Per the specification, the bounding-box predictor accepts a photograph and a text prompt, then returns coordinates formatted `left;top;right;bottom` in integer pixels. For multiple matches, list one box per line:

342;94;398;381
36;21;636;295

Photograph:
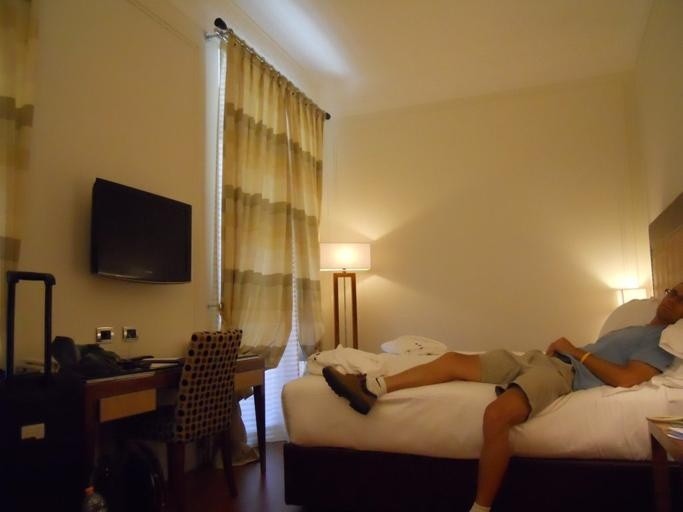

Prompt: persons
323;279;682;512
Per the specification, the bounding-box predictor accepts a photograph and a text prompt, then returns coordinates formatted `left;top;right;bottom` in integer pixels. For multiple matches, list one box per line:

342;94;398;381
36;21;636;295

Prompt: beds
281;193;683;512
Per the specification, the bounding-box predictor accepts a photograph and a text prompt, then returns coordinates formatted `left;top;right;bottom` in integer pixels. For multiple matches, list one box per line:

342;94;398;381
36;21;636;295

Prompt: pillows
600;296;683;389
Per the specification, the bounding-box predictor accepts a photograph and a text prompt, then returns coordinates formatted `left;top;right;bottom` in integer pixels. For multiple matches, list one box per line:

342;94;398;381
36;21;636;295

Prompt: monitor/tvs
90;178;192;285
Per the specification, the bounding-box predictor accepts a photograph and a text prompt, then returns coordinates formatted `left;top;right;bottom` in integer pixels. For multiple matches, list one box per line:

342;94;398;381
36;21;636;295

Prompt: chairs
113;328;242;512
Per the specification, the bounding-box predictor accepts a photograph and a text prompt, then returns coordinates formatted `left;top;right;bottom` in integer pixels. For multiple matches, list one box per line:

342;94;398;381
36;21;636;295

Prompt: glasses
665;288;683;304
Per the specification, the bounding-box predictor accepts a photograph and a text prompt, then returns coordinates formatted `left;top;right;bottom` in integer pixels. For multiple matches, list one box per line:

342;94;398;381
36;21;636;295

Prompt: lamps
319;243;371;349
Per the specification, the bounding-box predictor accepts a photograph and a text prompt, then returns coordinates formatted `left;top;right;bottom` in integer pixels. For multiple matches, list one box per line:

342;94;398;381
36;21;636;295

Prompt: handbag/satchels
79;342;150;375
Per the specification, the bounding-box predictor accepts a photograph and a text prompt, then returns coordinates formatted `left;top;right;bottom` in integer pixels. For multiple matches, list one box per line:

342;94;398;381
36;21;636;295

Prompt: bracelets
579;350;593;364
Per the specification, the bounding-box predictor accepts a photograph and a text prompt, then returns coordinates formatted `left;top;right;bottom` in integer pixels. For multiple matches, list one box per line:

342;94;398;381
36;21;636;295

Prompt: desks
0;358;269;512
648;414;683;512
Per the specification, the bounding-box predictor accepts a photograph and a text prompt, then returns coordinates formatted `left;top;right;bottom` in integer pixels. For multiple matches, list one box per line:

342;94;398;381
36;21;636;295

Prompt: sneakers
322;366;377;415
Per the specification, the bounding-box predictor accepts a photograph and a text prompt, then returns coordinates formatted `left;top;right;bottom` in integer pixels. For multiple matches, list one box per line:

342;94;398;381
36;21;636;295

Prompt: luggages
0;271;86;508
